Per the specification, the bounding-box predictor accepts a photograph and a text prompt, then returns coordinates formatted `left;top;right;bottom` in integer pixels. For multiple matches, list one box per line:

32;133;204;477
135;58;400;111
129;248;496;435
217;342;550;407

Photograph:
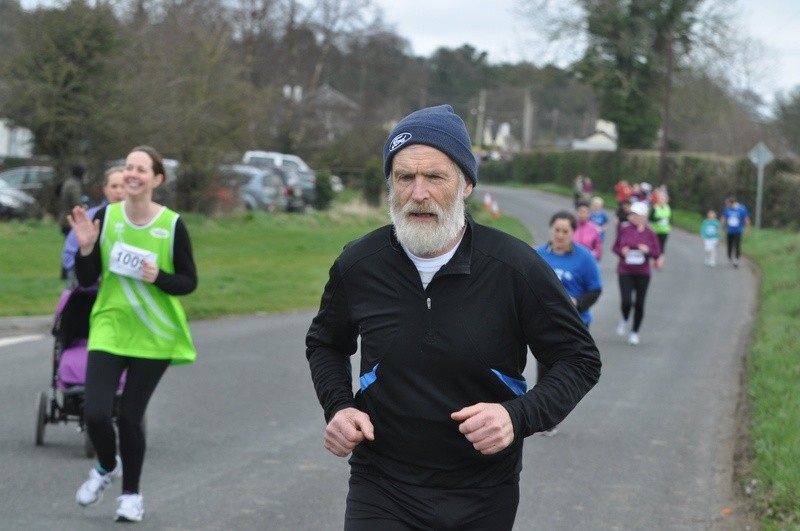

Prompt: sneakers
75;457;122;506
113;494;146;522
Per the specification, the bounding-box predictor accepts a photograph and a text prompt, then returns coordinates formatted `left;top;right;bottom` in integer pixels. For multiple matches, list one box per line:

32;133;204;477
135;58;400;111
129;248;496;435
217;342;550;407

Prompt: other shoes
629;331;638;345
617;318;629;336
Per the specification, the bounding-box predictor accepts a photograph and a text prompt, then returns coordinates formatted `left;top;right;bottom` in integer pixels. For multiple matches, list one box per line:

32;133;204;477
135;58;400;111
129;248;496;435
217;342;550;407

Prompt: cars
110;160;178;203
0;179;35;216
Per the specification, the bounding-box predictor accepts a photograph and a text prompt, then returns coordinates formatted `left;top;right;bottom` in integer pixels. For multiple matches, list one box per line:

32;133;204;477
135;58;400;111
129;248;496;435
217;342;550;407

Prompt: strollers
36;287;126;445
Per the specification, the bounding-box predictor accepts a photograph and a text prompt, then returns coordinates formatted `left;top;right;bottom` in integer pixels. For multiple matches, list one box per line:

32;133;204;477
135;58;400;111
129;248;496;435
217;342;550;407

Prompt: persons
720;195;750;268
305;103;602;531
61;146;198;520
532;175;609;333
614;178;672;271
611;202;661;345
700;210;721;268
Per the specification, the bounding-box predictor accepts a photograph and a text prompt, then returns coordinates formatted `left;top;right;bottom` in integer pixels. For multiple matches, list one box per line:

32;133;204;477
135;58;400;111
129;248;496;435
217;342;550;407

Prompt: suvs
216;148;344;213
0;166;55;198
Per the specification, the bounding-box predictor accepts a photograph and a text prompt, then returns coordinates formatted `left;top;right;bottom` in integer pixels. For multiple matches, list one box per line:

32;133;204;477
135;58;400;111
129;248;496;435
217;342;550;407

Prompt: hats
629;202;649;217
381;103;479;188
